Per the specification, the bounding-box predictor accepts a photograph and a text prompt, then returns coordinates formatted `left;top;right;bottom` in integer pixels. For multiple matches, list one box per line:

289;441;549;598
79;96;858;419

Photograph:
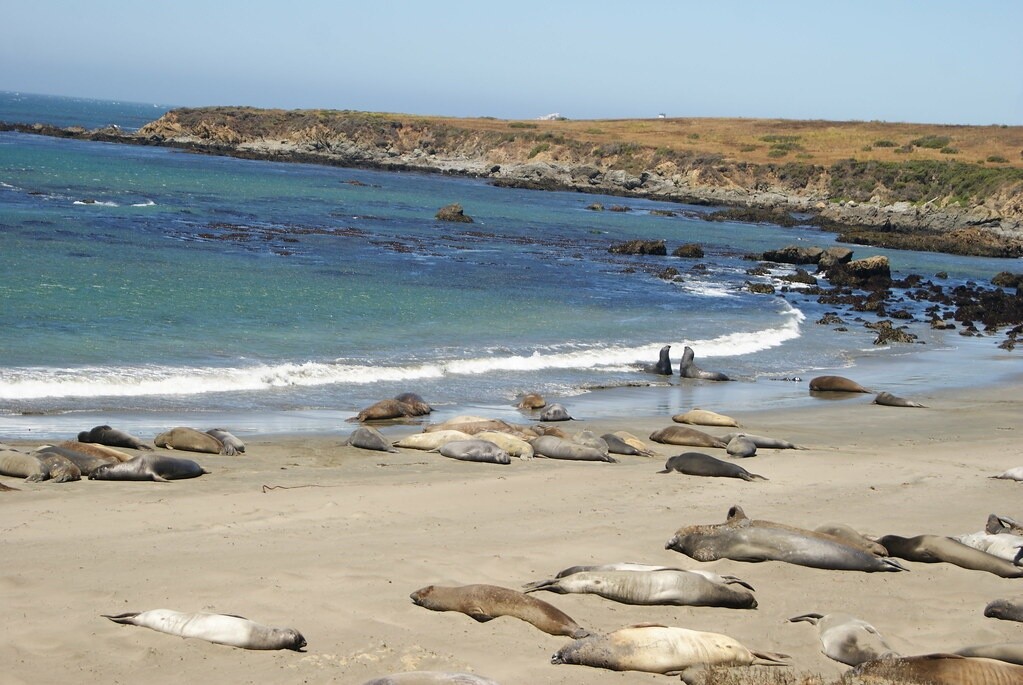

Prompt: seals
97;609;308;651
809;376;874;395
347;391;1023;685
643;346;673;375
1;422;246;485
680;346;736;382
874;392;930;408
